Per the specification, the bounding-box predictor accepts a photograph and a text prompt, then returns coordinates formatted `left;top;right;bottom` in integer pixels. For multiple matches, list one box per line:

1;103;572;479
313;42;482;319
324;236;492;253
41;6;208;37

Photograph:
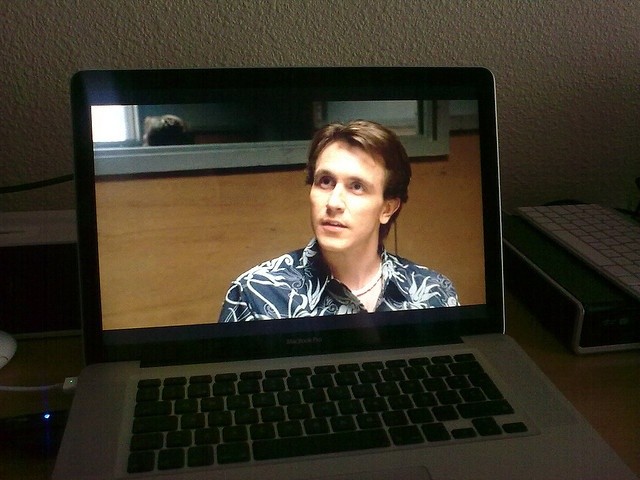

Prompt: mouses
0;330;20;372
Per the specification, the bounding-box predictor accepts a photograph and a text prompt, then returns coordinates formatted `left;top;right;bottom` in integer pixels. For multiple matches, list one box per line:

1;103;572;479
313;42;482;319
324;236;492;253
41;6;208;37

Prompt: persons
217;117;463;323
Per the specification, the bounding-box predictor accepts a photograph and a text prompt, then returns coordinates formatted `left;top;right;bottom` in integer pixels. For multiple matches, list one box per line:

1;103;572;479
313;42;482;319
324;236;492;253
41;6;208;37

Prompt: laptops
51;67;640;479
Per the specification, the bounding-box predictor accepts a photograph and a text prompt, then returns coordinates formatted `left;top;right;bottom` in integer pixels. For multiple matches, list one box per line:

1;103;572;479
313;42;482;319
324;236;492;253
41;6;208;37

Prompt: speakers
0;209;83;337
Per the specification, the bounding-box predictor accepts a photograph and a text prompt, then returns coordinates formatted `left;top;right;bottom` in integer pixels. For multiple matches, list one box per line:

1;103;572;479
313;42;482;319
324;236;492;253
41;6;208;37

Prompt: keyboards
516;202;639;301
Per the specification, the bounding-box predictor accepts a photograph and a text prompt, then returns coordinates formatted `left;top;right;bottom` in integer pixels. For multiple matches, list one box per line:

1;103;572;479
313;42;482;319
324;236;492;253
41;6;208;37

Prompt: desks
0;286;640;479
